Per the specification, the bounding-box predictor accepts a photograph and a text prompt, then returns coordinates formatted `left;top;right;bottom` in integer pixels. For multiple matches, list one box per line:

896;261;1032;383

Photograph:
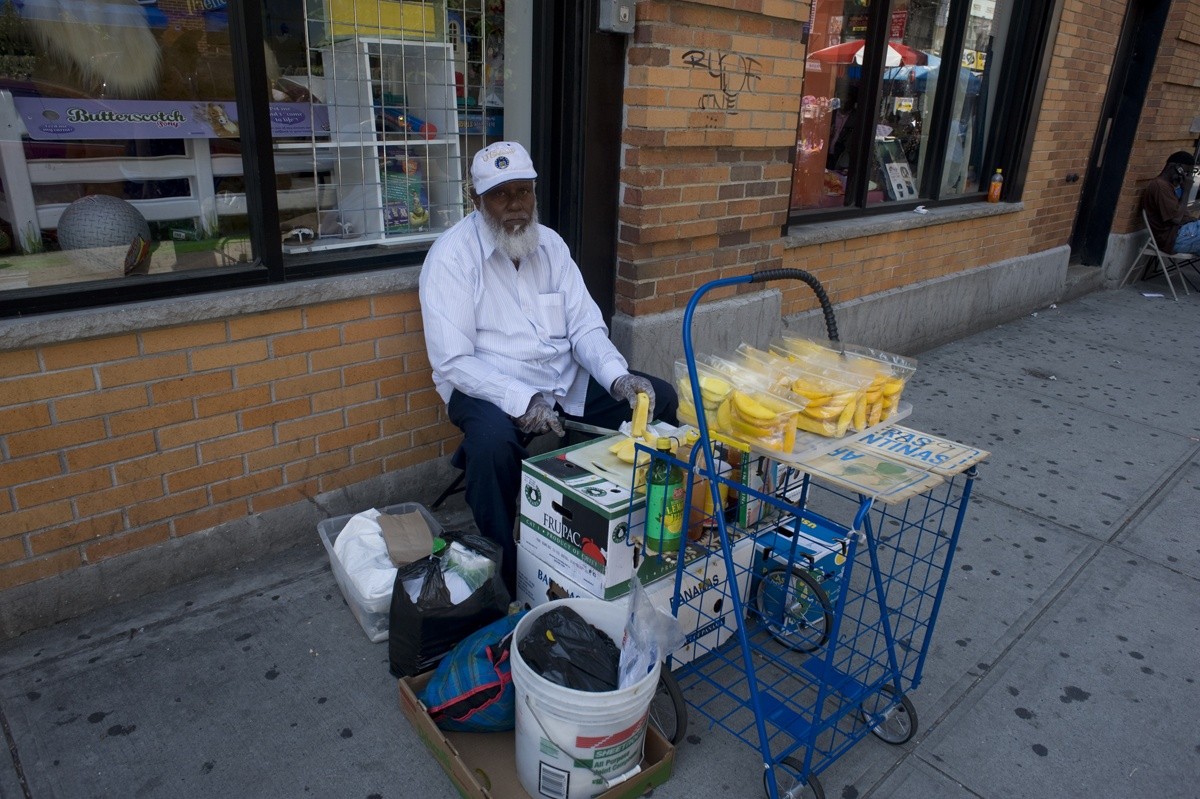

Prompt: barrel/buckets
509;598;662;799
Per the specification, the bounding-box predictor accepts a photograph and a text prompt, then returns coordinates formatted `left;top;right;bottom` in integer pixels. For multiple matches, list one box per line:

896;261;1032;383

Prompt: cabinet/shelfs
319;38;464;240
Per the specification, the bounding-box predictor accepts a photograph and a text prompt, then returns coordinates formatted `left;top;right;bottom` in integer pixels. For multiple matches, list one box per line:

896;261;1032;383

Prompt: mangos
611;338;902;464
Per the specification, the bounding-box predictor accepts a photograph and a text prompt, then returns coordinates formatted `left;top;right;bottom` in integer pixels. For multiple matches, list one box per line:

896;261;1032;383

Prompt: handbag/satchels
417;608;530;733
388;530;512;677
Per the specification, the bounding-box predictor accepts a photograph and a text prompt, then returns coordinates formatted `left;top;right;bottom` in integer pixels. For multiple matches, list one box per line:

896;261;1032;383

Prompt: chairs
1118;189;1200;301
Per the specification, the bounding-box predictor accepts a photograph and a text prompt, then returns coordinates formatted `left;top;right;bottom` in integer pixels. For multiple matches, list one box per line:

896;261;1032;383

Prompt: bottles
701;458;732;527
677;433;709;540
988;168;1003;203
644;437;688;553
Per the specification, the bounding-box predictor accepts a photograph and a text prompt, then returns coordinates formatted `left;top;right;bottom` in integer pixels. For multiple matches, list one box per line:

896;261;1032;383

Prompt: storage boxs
317;419;855;799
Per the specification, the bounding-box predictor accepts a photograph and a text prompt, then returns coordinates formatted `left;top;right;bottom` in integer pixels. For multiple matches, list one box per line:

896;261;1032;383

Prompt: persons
895;111;922;163
420;142;680;596
1141;151;1200;254
942;112;964;186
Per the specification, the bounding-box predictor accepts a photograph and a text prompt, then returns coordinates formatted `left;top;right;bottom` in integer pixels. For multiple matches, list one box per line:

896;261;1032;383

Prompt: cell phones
1176;166;1186;176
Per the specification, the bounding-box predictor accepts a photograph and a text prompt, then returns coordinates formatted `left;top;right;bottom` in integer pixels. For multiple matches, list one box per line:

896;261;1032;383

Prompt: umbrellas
846;51;981;110
807;40;928;124
